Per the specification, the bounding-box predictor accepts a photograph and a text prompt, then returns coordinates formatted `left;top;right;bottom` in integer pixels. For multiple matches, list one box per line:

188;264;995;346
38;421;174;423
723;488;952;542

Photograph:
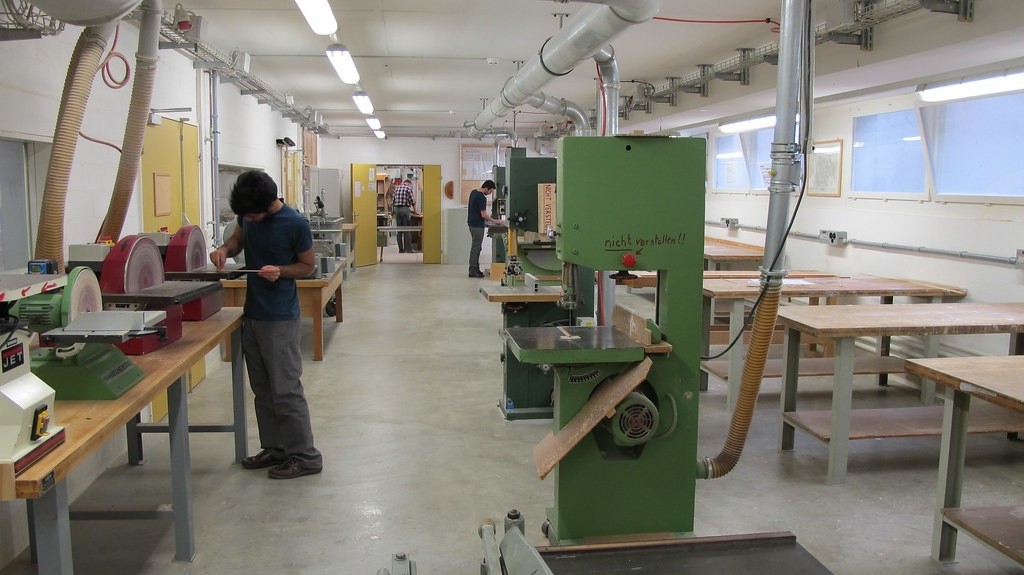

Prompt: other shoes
407;249;417;253
399;250;406;253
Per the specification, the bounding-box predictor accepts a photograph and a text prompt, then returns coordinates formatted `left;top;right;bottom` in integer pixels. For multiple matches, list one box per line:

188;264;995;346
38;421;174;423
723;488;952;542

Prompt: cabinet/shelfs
376;175;389;212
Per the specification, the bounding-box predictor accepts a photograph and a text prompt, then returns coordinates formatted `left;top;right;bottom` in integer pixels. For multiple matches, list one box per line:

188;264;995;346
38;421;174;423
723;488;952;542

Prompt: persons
210;171;323;480
466;180;506;278
394;179;418;255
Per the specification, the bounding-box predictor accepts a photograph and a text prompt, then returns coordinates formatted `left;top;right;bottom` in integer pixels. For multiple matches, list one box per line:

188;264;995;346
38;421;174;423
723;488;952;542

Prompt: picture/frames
806;139;843;197
154;173;171;217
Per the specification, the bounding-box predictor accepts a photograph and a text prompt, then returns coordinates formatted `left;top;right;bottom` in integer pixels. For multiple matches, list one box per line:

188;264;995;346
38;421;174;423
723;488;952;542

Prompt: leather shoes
268;461;322;480
241;450;287;469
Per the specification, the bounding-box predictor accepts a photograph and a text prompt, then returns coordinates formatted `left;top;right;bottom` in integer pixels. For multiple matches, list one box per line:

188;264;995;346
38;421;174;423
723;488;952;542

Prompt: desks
220;257;345;362
703;278;967;416
342;223;359;280
704;240;765;325
0;307;248;575
630;270;840;358
775;304;1024;486
411;213;423;249
377;226;423;264
905;355;1023;567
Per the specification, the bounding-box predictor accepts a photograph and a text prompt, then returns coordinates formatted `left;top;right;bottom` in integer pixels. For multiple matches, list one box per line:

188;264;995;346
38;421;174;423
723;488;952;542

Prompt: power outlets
725;218;738;230
828;231;847;246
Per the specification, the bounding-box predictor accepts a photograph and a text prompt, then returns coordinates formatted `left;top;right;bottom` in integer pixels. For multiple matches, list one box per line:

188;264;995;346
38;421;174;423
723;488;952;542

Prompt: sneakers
469;272;484;277
478;270;483;275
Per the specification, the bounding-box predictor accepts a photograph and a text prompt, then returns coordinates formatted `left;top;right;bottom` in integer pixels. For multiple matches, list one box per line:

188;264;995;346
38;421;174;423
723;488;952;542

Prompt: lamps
717;111;800;134
295;0;385;139
915;66;1024;102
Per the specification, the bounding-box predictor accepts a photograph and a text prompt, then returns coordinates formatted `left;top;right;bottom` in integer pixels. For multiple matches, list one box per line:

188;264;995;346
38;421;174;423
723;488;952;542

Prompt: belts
394;205;411;207
